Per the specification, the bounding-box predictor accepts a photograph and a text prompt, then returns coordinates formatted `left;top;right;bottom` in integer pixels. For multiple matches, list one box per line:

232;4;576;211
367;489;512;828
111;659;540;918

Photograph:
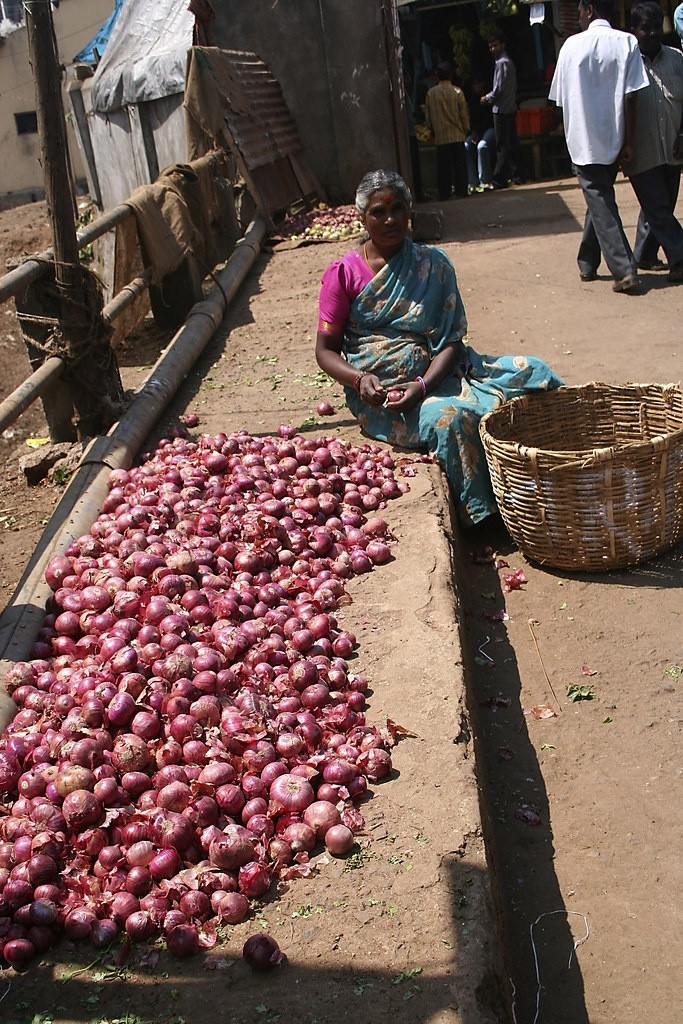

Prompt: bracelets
354;371;374;393
414;375;428;400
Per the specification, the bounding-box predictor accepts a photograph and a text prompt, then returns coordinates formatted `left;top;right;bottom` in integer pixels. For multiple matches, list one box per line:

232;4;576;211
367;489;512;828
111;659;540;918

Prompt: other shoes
467;182;492;195
667;271;683;282
612;273;641;292
578;271;597;280
638;259;669;270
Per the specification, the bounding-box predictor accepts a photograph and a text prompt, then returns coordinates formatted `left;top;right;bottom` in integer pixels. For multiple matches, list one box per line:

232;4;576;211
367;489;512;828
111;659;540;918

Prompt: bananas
502;2;519;17
413;123;430;141
449;23;476;75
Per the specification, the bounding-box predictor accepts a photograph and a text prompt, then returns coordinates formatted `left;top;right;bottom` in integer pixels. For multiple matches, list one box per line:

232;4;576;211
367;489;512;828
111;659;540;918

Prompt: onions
388;390;403;402
0;402;401;967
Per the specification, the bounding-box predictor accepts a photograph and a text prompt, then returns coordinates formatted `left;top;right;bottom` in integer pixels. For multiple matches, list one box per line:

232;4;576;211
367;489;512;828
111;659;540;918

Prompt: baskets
479;379;683;573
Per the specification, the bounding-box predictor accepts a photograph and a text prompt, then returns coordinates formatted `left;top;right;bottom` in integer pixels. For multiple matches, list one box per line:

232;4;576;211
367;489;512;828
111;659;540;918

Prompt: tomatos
279;205;366;239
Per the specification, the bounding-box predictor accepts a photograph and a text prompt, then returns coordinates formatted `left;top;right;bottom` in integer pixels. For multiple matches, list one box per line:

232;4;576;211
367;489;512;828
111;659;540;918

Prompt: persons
314;171;571;566
673;3;683;52
424;62;471;201
464;76;498;196
548;0;645;293
623;4;683;281
478;34;525;187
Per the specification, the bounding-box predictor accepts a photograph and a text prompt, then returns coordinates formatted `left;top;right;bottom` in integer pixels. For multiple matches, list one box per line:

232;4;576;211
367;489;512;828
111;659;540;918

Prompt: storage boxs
514;103;557;138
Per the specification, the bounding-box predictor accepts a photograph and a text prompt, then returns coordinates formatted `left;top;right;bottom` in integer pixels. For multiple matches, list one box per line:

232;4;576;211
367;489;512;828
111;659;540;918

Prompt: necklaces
357;237;401;277
